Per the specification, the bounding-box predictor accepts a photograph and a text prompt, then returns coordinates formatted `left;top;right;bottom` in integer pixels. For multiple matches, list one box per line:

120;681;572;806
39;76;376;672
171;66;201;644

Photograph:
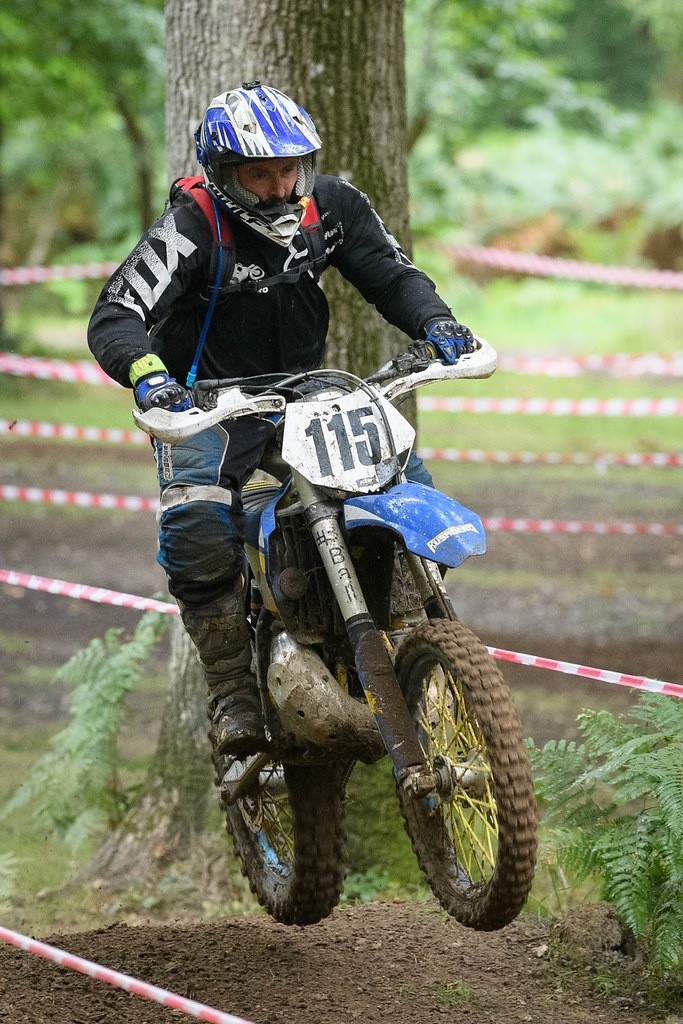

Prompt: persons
86;82;476;755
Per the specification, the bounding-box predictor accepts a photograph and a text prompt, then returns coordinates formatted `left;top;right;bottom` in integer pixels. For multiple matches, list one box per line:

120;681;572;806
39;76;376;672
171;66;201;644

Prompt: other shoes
207;677;264;751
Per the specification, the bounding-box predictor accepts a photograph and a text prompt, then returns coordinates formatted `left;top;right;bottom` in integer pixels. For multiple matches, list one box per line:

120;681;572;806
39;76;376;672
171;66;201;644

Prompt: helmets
195;82;322;245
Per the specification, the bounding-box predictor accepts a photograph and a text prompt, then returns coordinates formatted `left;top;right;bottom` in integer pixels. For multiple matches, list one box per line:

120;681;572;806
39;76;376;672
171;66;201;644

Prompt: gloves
424;316;477;366
135;373;192;414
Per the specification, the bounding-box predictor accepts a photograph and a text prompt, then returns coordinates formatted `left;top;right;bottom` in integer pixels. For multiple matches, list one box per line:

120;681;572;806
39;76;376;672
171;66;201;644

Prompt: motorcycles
131;335;539;931
